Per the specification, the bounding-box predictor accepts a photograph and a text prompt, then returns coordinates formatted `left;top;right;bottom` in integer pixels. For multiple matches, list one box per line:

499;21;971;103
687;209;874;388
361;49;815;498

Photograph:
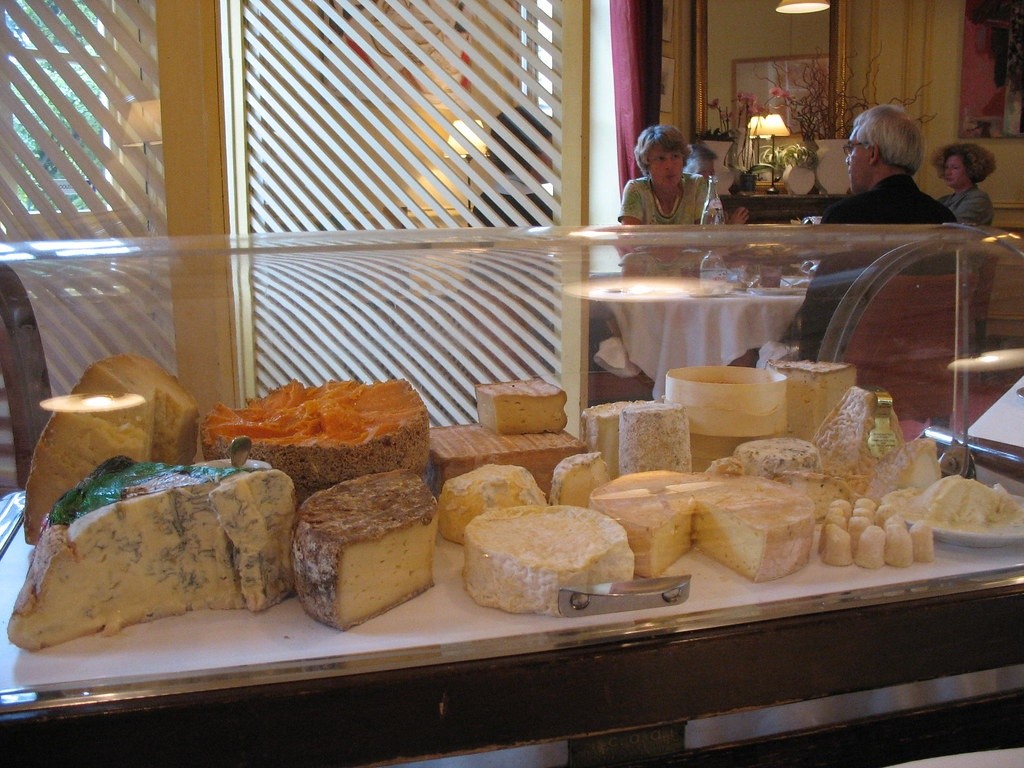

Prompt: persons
685;142;719;183
618;124;749;278
799;106;959;363
929;141;997;226
468;105;617;371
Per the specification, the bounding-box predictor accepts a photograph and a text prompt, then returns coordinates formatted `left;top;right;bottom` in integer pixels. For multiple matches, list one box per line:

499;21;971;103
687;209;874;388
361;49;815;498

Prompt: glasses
843;140;871;155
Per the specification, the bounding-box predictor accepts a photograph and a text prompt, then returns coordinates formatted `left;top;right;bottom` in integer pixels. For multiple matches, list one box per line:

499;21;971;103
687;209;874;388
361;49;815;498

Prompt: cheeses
5;354;941;653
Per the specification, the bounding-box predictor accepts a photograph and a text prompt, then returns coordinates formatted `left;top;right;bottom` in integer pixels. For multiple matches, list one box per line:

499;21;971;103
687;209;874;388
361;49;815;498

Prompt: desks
564;280;807;400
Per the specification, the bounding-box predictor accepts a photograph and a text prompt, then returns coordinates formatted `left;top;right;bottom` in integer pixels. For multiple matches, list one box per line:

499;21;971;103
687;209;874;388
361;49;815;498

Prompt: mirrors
693;0;844;194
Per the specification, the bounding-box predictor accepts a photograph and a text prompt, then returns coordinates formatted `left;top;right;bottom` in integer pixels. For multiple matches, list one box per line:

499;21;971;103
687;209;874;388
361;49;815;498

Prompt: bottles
699;174;724;225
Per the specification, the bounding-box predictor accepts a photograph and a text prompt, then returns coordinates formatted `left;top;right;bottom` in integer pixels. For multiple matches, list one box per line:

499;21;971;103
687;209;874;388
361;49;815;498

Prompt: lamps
444;120;488;223
124;100;162;146
775;0;830;15
747;116;768;182
762;113;791;193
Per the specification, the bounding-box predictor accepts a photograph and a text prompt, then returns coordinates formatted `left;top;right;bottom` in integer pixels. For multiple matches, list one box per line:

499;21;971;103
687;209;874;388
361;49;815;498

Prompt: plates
751;279;810;295
880;489;1024;549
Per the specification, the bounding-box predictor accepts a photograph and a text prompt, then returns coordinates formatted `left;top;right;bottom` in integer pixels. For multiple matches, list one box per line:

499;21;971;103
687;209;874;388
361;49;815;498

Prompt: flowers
707;87;793;172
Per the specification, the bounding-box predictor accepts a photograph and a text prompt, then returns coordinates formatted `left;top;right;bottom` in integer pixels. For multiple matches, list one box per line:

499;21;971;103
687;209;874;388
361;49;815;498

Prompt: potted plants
781;144;818;195
761;145;787;182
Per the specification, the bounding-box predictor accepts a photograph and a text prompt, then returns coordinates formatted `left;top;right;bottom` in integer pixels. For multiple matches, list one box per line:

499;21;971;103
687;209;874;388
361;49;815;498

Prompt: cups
758;266;783;288
